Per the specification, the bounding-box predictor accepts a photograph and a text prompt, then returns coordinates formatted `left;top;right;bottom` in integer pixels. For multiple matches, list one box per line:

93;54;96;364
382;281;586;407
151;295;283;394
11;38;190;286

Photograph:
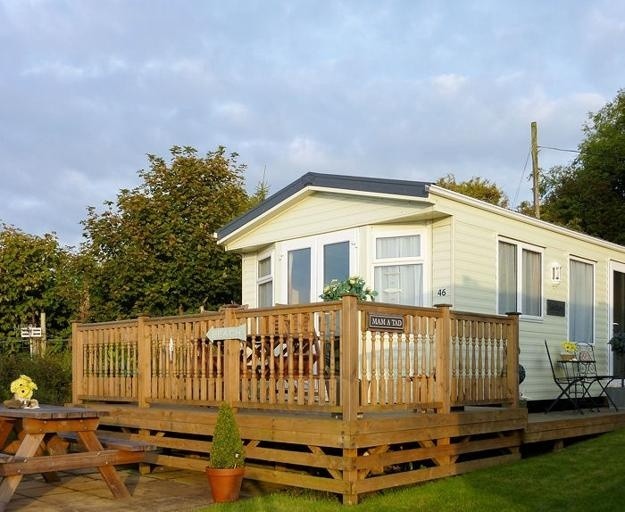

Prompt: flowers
10;375;38;404
320;275;380;303
561;342;576;353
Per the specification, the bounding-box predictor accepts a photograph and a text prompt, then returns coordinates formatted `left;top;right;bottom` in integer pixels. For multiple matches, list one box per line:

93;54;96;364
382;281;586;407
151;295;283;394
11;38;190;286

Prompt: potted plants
206;400;246;503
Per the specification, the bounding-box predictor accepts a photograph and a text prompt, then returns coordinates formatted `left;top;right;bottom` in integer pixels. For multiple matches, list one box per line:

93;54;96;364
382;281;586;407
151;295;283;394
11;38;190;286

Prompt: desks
1;403;153;512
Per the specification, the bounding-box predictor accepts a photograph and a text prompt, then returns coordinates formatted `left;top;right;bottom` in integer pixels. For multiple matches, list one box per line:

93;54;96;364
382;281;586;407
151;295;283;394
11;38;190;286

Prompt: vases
559;354;577;361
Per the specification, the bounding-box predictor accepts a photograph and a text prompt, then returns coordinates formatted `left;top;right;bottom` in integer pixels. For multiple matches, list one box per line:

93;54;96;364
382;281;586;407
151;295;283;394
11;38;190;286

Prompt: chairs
544;339;594;416
576;340;620;413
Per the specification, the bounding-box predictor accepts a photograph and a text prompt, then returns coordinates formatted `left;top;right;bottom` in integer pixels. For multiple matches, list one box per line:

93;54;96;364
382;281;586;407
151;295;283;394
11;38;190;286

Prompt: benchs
56;431;156;451
